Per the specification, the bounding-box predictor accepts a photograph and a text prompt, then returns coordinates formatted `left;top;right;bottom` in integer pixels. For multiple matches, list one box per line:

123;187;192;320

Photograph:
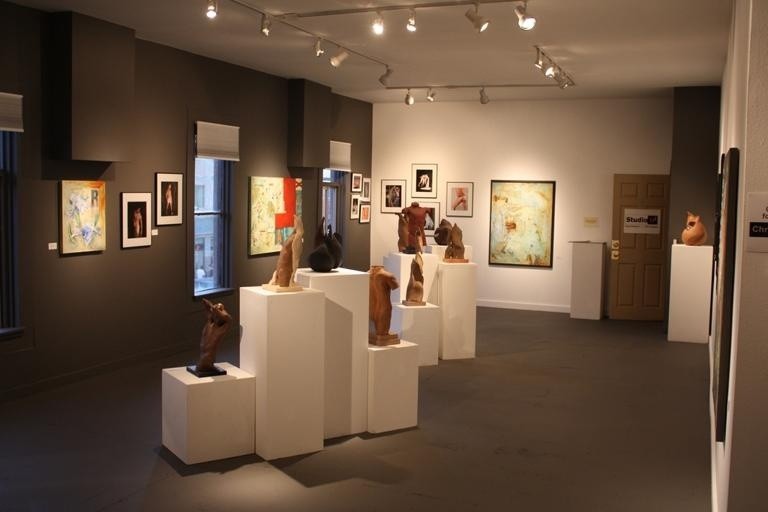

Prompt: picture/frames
711;149;741;440
350;194;360;219
411;163;438;198
155;172;183;226
58;179;107;256
381;179;407;214
359;204;371;224
362;178;371;202
446;181;474;217
121;191;152;248
490;180;555;268
351;172;363;192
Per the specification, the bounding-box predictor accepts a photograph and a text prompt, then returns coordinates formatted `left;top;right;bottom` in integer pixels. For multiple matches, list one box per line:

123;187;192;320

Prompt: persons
198;297;233;370
418;174;429;188
452;187;467;210
165;183;173;216
402;202;433;245
132;206;143;238
390;187;399;206
366;265;399;336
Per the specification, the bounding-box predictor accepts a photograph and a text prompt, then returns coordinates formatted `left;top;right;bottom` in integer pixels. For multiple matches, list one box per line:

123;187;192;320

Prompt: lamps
370;16;385;35
314;39;323;58
405;89;414;104
260;16;272;36
380;68;393;86
427;88;436;101
534;47;569;90
328;48;348;67
464;9;489;32
407;13;417;33
514;6;536;31
479;89;491;104
205;1;218;19
